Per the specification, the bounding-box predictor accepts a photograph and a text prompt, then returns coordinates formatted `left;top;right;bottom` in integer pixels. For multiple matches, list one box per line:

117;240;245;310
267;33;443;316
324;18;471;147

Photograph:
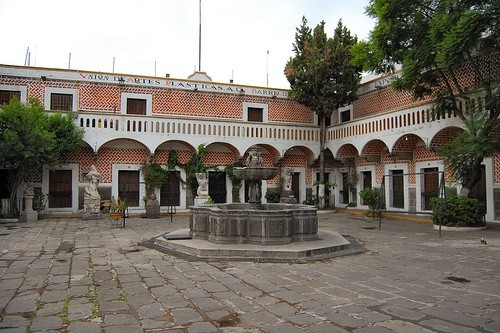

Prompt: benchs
81;199;111;209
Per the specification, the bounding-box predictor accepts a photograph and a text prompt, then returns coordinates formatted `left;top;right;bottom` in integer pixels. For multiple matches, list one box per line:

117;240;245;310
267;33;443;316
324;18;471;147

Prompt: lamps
402;135;408;141
290;147;294;150
